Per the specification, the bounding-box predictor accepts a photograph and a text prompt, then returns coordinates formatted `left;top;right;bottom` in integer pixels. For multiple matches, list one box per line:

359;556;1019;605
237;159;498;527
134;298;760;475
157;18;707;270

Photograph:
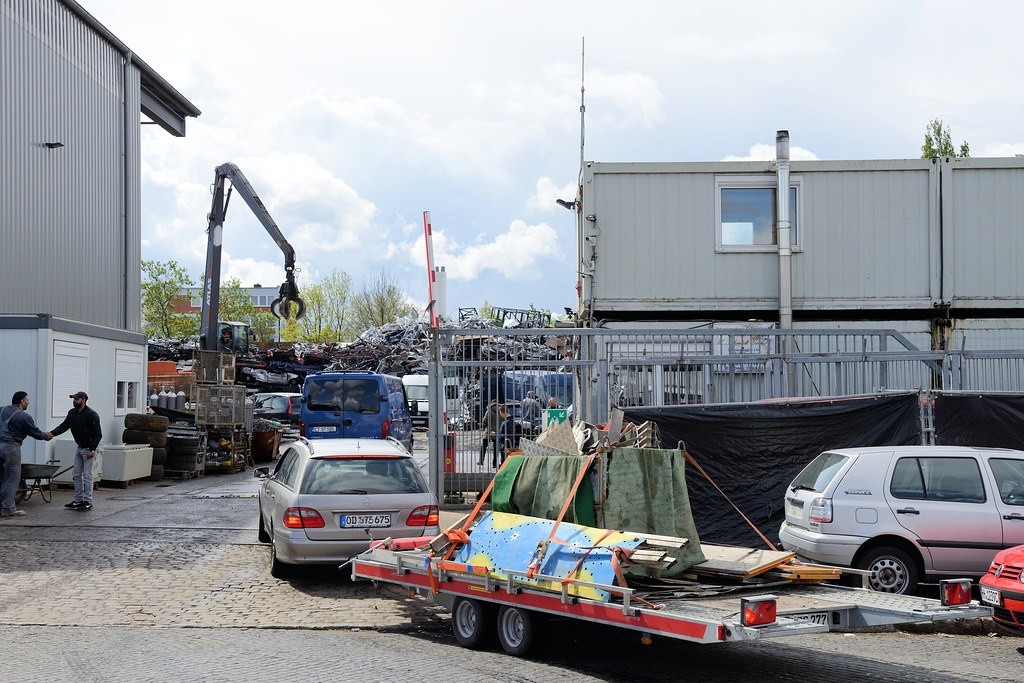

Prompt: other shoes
1;510;26;517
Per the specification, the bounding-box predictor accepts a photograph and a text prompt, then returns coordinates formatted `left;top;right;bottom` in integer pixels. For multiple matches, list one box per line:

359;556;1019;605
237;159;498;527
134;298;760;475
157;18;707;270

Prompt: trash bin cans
251;428;280;463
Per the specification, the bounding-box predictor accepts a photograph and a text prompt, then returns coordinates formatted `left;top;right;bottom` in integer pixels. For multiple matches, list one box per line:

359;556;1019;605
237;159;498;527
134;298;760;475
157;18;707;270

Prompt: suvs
236;391;302;429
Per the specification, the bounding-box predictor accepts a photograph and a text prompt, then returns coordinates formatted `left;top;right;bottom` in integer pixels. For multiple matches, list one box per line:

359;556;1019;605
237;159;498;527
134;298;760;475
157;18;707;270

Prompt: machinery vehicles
200;159;304;392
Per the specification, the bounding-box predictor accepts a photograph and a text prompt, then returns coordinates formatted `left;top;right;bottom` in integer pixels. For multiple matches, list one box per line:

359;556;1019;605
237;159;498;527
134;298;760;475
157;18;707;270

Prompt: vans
481;370;581;423
778;445;1024;596
398;373;461;431
301;369;420;460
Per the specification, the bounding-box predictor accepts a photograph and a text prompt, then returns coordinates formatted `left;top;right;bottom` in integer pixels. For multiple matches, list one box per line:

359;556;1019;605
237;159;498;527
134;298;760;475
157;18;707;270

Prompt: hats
69;392;88;402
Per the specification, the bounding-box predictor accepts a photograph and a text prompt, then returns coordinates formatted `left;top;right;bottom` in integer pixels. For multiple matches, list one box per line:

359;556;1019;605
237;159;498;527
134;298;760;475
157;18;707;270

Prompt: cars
978;543;1024;640
252;434;441;583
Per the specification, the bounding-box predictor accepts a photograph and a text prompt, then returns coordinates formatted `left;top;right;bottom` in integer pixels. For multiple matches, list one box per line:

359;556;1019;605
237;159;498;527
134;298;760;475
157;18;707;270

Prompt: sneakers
78;501;92;512
64;500;84;509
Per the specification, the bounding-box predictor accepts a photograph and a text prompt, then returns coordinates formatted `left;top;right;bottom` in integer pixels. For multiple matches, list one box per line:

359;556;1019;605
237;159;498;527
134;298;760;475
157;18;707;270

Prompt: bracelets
89;449;95;453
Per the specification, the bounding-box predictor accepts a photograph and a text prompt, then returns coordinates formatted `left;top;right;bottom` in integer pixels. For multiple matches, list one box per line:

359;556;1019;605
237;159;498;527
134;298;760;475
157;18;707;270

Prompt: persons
492;405;513;466
50;392;102;512
221;329;231;344
520;391;540;423
0;391;54;516
477;399;504;468
548;398;563;409
